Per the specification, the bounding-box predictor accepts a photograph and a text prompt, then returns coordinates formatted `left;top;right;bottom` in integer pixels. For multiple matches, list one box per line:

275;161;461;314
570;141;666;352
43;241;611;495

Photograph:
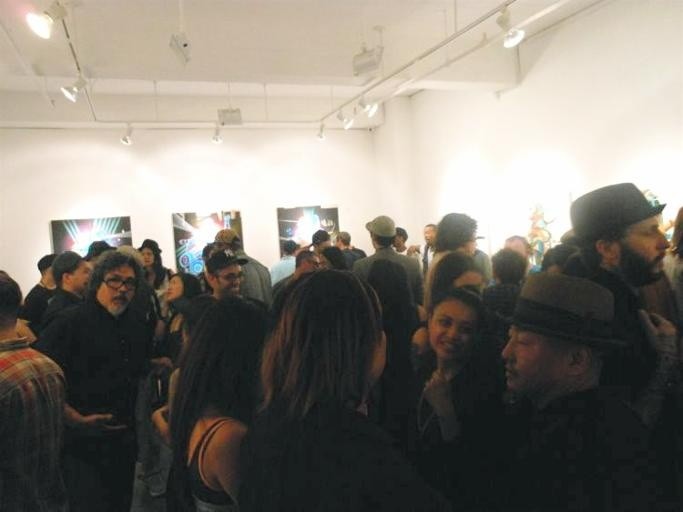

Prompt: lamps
25;4;87;103
114;122;327;148
337;10;525;130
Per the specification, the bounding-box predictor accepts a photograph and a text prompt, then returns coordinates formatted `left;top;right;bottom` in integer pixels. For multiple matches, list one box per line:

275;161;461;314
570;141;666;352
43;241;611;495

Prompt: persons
2;178;682;510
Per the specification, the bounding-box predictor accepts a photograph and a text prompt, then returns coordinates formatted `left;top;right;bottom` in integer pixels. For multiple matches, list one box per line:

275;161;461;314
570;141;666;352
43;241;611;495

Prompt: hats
138;240;162;254
213;228;241;245
82;239;117;262
365;215;396;238
37;253;57;271
205;246;249;273
494;272;634;350
52;249;80;272
560;186;666;245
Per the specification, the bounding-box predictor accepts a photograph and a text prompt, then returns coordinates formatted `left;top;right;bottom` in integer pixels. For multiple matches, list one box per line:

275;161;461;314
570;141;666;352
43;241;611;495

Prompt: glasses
99;276;136;292
306;259;320;268
218;271;244;281
